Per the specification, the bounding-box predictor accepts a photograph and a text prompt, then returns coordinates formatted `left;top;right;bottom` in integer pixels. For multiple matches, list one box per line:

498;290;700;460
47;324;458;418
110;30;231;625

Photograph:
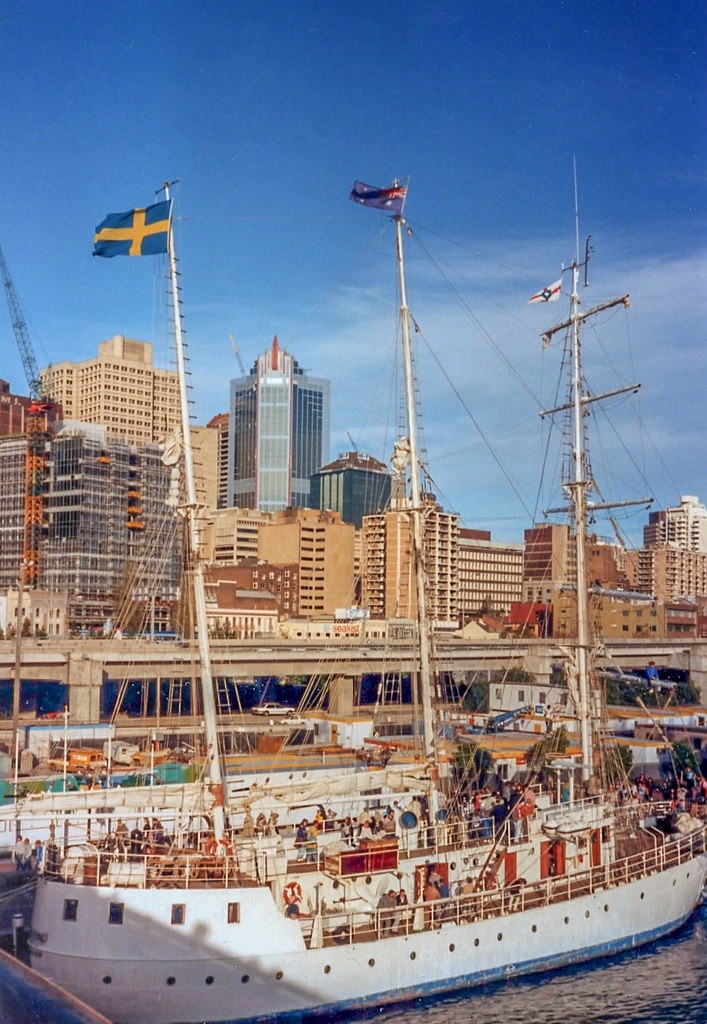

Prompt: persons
424;869;448;923
462;780;537;843
376;889;408;938
293;796;421;864
667;798;685;815
143;817;163;837
285;896;299;920
12;836;42;870
507;878;526;912
242;811;279;836
552;780;578;805
116;820;128;832
463;878;475;918
615;766;707;798
586;773;600;804
645;661;659;687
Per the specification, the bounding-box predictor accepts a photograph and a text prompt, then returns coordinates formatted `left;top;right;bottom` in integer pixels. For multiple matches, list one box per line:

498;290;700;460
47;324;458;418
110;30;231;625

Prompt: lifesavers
214;839;232;861
205;838;214;853
283;881;301;904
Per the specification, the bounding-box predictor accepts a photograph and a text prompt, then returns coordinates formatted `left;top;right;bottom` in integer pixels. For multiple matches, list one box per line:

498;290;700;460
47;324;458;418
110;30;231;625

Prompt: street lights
134;591;177;640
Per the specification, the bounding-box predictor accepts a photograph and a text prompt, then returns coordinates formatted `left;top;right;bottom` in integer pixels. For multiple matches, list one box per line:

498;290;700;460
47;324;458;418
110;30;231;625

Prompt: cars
250;702;296;716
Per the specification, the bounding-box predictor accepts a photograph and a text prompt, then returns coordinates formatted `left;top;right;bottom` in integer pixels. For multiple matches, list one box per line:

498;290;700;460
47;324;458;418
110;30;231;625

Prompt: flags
348;180;407;209
91;198;173;256
528;279;560;304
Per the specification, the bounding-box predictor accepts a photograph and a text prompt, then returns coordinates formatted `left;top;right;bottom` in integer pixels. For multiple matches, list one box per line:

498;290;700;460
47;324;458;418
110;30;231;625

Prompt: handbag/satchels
293;835;302;850
309;832;317;841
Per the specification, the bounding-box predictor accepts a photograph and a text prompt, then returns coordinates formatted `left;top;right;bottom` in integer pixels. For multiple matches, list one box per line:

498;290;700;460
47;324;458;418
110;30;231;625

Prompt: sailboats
0;153;707;1024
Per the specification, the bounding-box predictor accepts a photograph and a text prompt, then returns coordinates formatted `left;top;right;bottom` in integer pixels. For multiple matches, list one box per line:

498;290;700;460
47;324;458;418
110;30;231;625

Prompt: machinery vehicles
464;705;533;735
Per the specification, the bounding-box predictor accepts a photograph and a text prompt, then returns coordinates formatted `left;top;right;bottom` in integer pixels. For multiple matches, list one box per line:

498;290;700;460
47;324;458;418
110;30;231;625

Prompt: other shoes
392;932;400;935
380;933;389;938
